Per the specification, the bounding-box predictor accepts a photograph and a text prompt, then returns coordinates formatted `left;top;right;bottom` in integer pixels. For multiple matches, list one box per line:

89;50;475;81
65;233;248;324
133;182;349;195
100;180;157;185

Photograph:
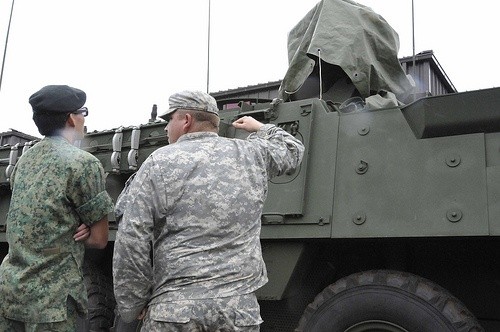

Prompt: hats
28;84;88;113
157;89;219;121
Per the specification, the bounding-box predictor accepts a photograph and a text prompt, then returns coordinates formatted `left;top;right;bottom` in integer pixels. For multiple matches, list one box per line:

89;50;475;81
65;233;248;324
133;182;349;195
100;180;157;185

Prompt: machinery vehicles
0;87;500;332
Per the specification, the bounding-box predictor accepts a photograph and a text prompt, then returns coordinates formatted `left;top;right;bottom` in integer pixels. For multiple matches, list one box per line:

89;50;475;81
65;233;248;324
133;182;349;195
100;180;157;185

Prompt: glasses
52;106;89;117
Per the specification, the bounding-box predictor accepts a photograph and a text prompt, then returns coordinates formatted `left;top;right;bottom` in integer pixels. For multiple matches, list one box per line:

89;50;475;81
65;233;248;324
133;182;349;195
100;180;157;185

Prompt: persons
0;85;115;332
113;89;305;332
